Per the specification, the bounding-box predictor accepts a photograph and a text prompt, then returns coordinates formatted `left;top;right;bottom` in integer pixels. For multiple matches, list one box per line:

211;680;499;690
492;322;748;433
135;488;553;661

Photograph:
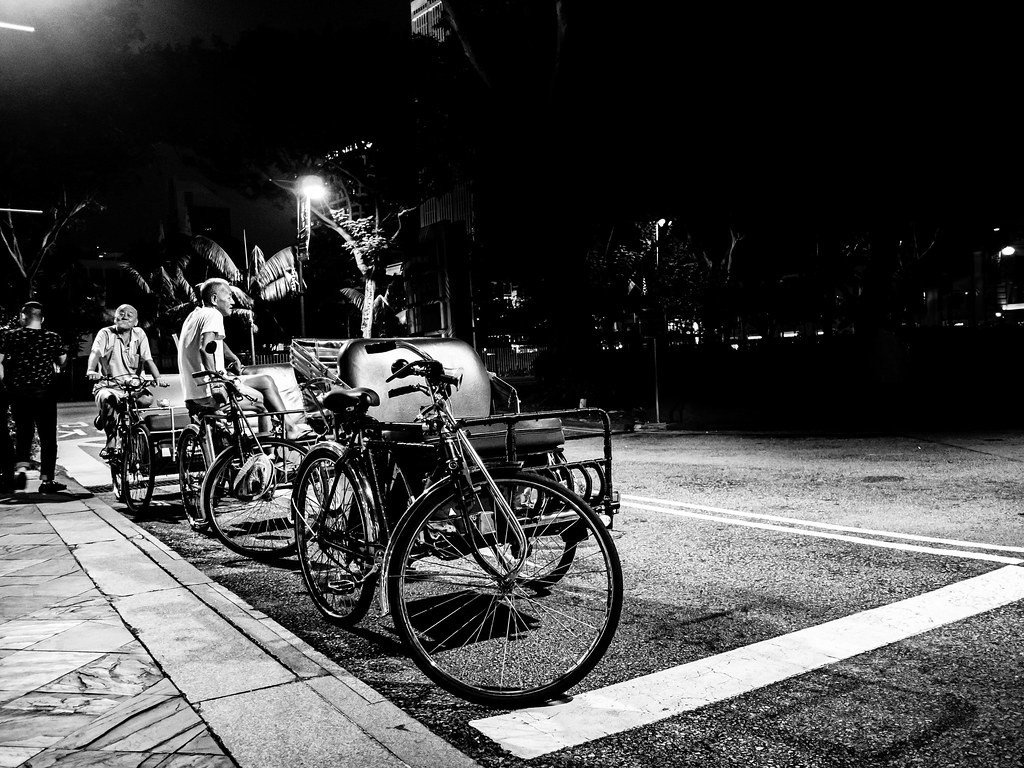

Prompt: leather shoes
15;472;27;489
39;481;67;493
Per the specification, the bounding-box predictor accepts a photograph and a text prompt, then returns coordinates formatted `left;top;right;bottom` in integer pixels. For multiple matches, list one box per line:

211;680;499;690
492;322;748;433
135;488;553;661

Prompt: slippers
295;423;313;439
273;460;300;473
99;447;116;458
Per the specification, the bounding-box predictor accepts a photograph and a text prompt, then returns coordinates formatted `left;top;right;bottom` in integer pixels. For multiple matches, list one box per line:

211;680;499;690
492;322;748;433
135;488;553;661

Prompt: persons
0;301;70;493
176;276;312;464
85;303;170;460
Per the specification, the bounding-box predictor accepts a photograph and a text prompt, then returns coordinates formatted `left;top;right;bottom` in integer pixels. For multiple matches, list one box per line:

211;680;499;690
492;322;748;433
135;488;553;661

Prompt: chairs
338;336;566;454
230;363;317;441
142;374;194;427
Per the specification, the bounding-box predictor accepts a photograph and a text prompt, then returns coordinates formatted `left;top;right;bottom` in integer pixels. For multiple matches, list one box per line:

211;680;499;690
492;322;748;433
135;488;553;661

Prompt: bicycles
85;331;627;708
